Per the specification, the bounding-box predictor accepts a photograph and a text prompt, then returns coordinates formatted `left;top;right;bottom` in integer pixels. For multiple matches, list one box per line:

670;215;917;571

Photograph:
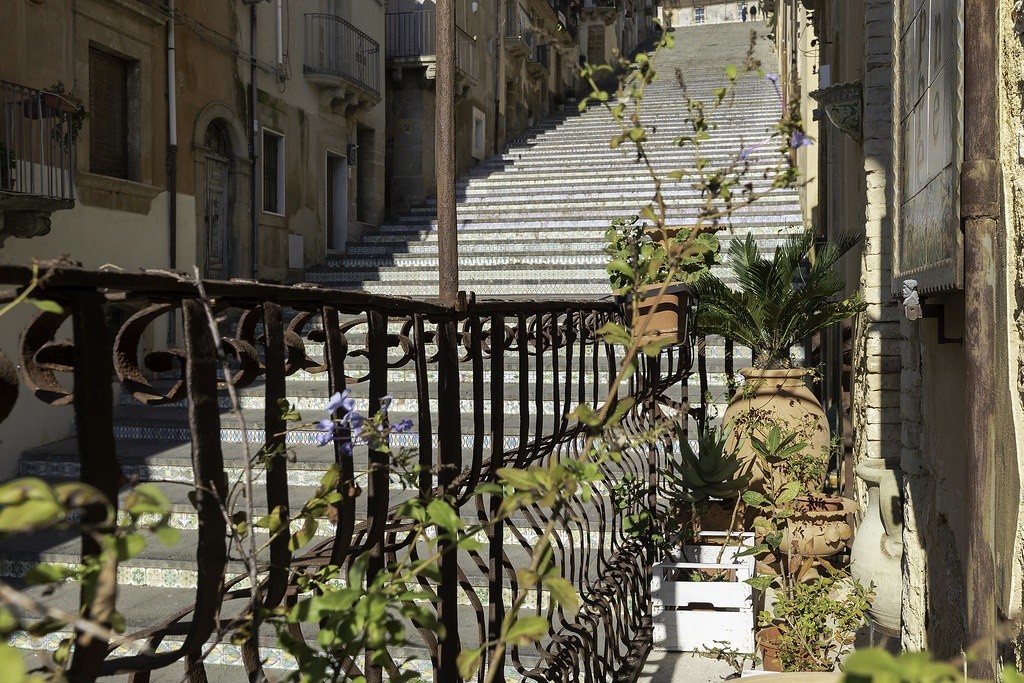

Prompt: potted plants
609;214;721;350
644;257;878;683
18;79;90;153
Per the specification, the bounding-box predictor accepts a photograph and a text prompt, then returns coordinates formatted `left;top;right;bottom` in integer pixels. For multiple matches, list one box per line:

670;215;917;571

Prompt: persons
742;7;748;22
750;4;757;21
